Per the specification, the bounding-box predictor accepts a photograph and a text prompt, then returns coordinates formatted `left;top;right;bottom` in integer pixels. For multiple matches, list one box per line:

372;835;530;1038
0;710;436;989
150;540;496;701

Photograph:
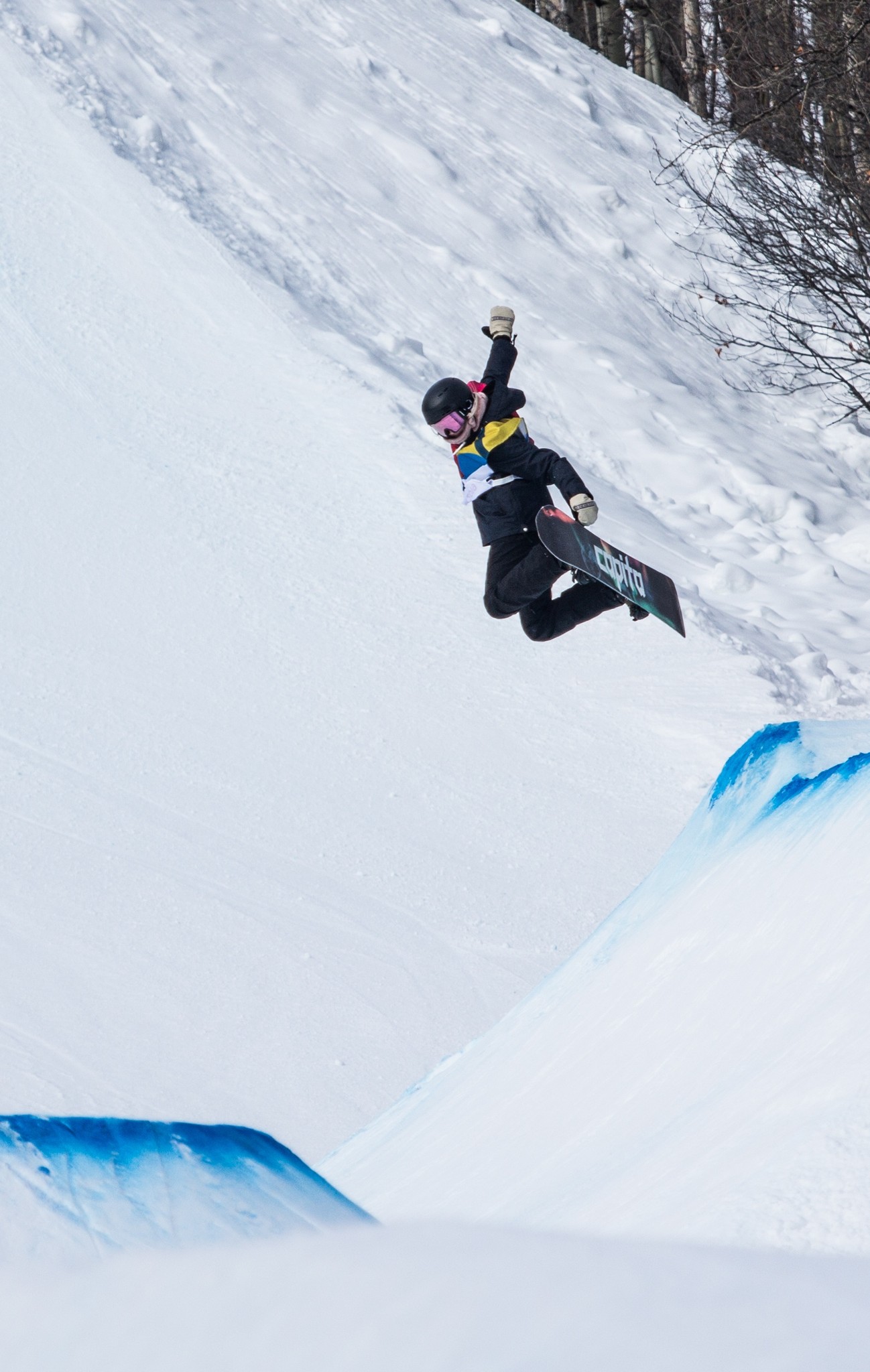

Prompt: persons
422;305;649;642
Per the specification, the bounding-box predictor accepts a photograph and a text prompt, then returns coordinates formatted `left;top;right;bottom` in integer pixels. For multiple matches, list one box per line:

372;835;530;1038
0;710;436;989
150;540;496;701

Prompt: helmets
422;377;474;440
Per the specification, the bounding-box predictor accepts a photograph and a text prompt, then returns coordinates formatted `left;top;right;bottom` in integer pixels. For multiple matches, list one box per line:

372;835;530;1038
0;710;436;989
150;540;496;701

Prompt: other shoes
560;563;596;585
616;593;649;621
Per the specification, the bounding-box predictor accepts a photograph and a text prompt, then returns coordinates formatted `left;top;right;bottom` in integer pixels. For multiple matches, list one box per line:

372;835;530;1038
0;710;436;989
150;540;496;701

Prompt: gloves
570;493;598;525
482;306;514;341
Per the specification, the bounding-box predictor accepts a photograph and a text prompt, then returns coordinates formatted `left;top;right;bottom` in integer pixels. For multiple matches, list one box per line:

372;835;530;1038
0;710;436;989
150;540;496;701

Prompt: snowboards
536;505;688;638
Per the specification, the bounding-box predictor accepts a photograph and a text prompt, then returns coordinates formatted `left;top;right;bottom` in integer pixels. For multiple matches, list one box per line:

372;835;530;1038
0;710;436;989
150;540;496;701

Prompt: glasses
431;410;465;437
